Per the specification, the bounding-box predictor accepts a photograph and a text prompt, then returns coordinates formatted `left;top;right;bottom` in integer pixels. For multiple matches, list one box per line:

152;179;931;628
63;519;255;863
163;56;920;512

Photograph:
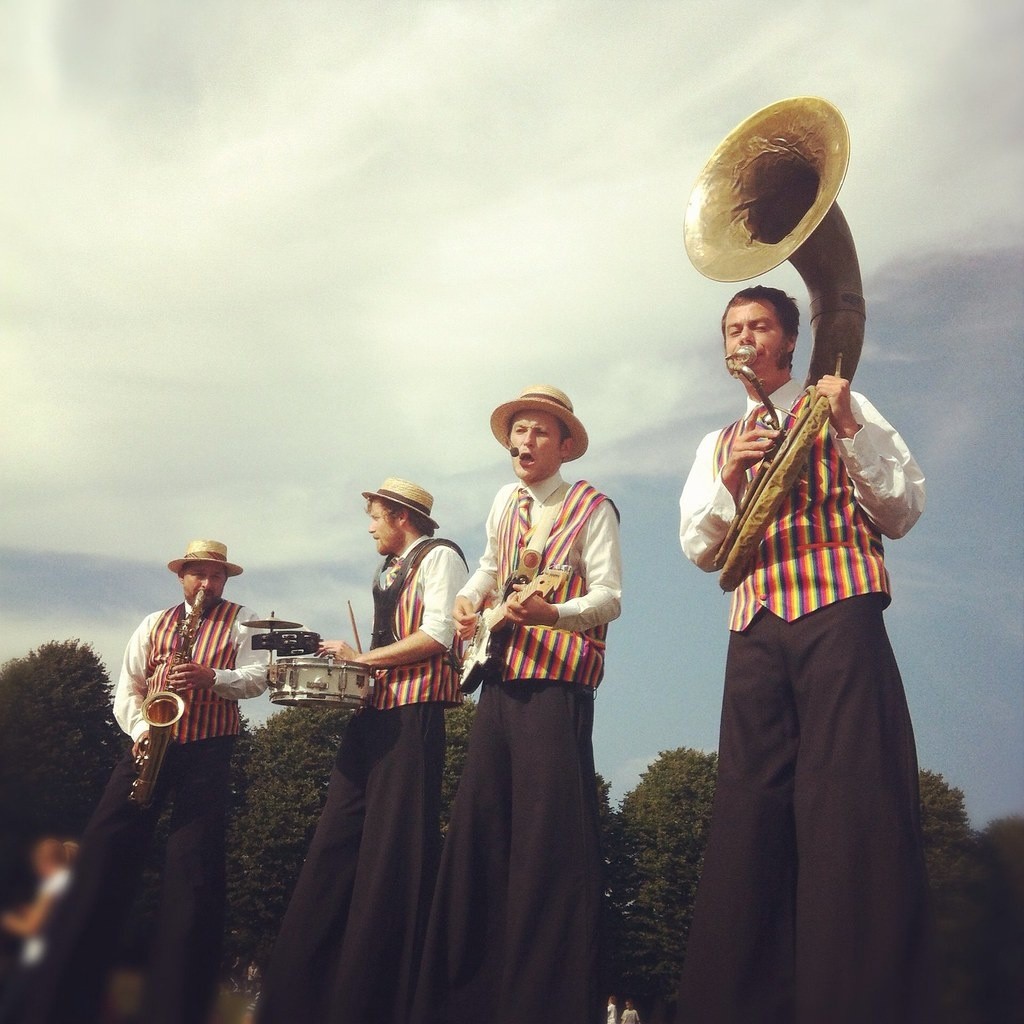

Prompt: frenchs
682;95;867;594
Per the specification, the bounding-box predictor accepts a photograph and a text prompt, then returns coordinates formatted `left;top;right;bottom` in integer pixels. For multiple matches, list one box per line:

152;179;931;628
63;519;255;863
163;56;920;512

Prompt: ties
744;403;774;486
386;558;405;589
511;490;535;572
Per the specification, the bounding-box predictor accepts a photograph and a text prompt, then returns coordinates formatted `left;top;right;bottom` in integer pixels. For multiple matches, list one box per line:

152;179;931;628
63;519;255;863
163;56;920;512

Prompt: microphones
504;436;519;459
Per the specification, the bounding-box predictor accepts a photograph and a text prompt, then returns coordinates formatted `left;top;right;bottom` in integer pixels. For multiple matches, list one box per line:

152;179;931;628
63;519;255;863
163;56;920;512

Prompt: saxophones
128;589;206;805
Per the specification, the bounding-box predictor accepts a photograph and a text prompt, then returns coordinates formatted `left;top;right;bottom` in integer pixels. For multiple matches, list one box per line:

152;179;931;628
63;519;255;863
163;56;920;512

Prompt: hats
167;539;244;577
361;478;439;530
490;385;588;464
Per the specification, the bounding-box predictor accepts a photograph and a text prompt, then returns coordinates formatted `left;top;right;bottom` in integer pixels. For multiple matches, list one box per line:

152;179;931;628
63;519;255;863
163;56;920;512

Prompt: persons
58;538;277;1022
453;379;628;1022
679;284;938;1023
248;476;472;1023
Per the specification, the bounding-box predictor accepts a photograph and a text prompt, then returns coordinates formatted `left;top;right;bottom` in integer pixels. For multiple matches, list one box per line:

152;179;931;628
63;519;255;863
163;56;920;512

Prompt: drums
267;657;370;710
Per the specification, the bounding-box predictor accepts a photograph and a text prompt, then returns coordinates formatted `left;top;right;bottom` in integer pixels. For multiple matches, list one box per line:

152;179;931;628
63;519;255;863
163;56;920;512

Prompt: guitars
457;563;574;695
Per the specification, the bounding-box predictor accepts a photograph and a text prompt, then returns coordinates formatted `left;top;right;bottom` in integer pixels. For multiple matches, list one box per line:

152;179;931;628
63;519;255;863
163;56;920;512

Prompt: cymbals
240;617;304;630
251;630;324;657
